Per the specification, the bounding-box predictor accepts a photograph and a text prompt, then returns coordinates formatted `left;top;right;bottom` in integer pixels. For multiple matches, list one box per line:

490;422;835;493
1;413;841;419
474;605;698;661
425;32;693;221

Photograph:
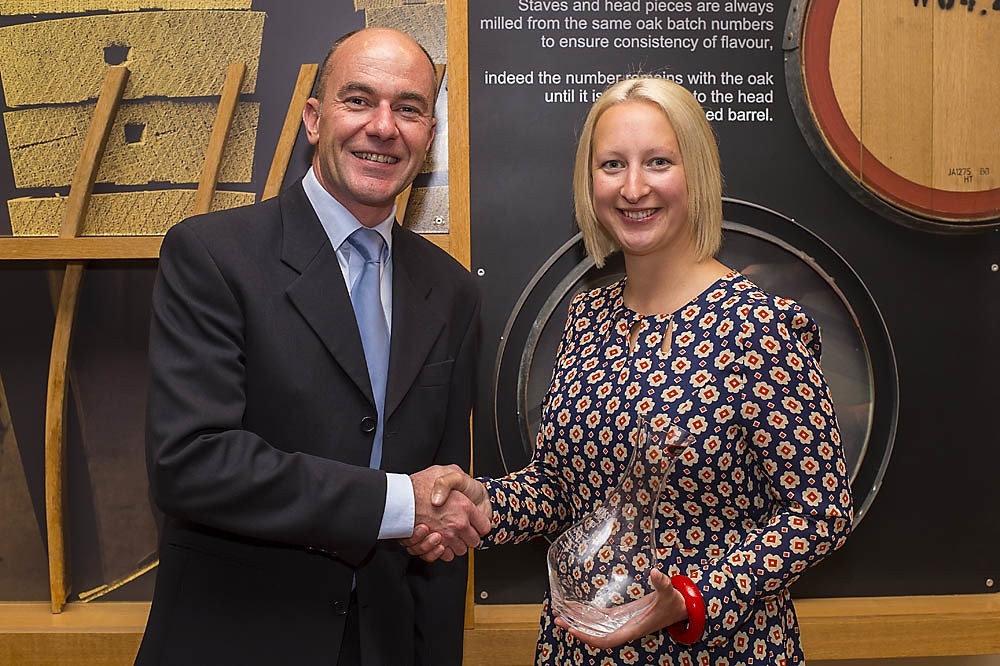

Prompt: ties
346;227;391;593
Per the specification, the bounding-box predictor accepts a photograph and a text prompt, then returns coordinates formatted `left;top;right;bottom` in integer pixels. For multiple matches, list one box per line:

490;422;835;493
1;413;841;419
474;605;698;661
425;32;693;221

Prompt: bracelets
666;575;705;646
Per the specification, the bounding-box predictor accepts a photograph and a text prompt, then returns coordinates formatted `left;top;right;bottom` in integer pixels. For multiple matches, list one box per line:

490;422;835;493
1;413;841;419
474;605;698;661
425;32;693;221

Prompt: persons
133;28;482;666
400;77;853;666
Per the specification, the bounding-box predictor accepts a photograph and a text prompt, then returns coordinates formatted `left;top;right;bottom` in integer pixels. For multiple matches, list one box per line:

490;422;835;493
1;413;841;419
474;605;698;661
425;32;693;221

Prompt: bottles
544;411;695;637
